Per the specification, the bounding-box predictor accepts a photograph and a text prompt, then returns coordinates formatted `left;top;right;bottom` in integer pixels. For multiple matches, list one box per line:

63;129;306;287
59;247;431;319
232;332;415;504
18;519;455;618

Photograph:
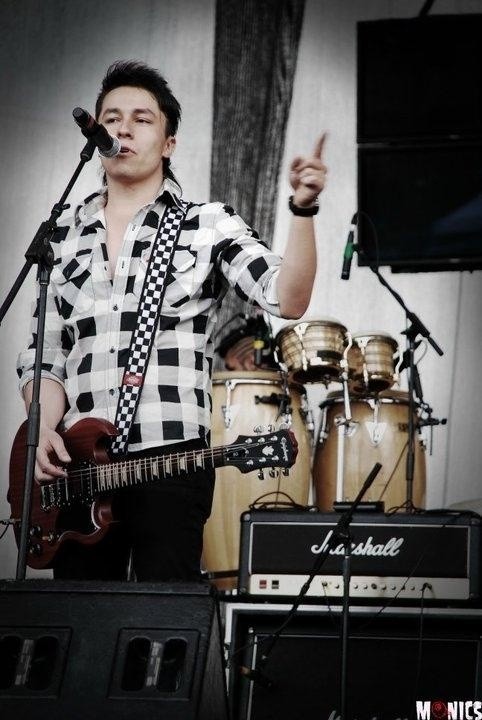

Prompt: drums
274;320;346;382
346;330;398;395
314;390;427;514
200;371;311;591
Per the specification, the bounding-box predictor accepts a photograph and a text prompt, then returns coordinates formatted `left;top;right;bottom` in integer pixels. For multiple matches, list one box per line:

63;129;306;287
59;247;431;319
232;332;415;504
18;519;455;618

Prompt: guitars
6;417;297;570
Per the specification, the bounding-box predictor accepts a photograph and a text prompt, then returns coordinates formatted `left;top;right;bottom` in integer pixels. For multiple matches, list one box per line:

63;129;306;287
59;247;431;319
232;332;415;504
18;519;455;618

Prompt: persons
16;59;328;580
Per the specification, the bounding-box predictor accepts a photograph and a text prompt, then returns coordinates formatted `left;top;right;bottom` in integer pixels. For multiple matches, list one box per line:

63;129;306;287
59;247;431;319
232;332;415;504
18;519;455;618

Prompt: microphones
340;212;359;281
72;107;122;161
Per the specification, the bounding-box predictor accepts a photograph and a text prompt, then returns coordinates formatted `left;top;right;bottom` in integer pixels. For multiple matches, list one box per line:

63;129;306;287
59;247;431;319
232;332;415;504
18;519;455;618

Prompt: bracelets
288;194;320;217
